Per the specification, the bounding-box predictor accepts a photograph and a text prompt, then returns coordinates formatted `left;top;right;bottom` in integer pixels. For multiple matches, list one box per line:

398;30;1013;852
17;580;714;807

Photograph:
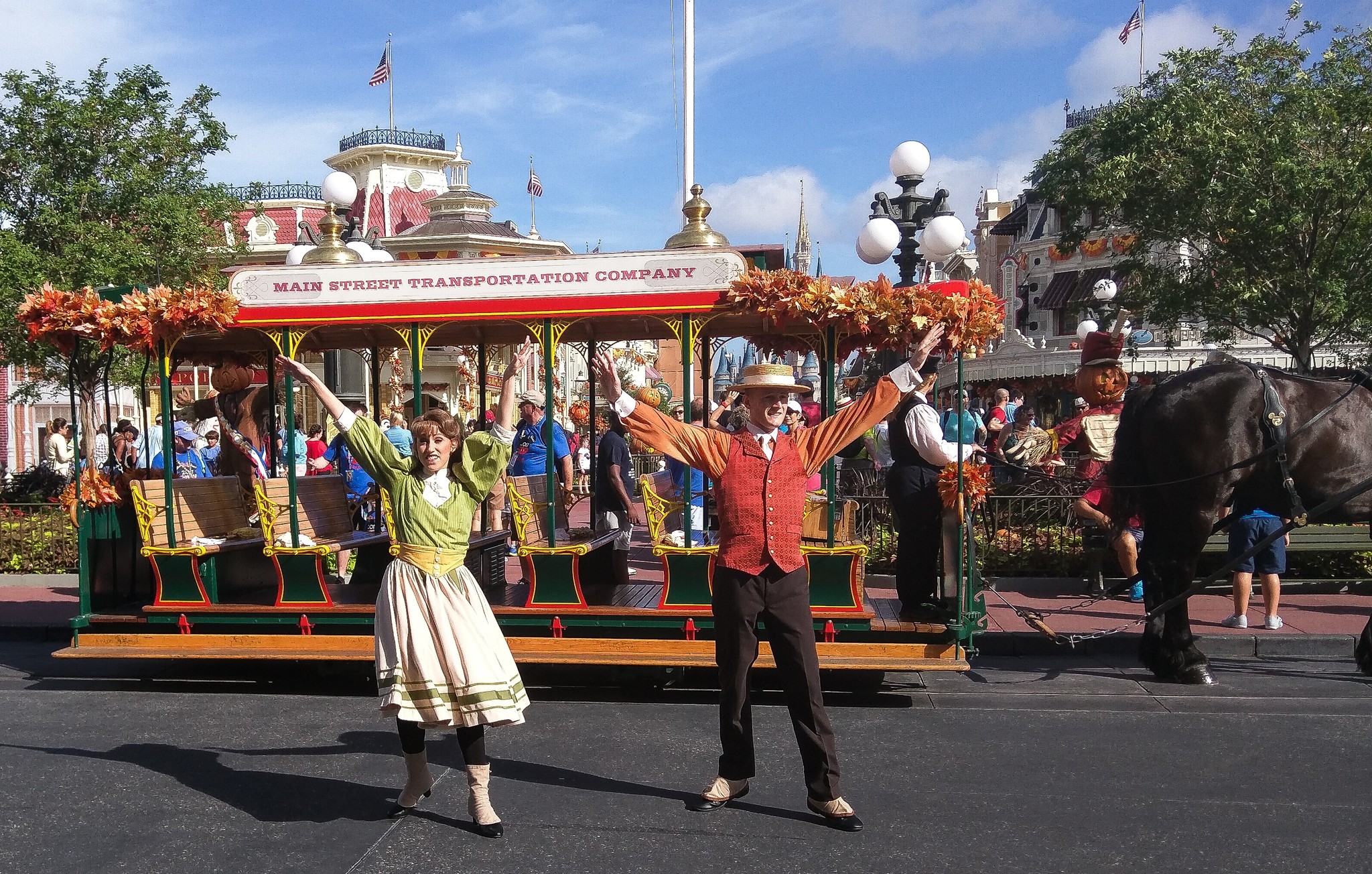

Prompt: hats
898;354;942;377
953;389;968;398
479;410;496;425
513;387;547;409
725;361;812;400
170;421;200;442
834;396;855;412
785;399;802;415
1074;396;1089;406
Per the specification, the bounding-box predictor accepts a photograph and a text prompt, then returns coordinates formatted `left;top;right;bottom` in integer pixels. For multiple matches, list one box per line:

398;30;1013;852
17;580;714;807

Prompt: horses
1103;352;1372;684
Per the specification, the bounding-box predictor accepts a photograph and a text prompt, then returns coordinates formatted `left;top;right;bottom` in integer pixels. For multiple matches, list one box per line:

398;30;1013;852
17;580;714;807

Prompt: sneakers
1129;578;1146;604
1221;613;1283;629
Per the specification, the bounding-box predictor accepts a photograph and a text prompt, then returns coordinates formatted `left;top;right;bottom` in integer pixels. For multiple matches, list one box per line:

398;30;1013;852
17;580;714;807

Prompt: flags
1119;6;1141;45
591;246;598;253
368;45;390;87
527;162;543;197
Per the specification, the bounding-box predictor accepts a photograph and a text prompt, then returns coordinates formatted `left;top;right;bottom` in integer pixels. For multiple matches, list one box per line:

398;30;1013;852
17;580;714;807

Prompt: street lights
855;139;963;283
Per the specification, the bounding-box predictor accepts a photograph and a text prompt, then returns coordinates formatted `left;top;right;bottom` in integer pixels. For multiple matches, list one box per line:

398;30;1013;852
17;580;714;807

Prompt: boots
387;746;432;820
464;755;504;839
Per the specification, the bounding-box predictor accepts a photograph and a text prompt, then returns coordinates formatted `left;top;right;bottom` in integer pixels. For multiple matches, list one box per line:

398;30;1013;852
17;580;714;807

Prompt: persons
44;385;1092;540
1073;473;1145;603
589;321;945;832
885;355;987;625
1218;501;1290;629
271;337;533;838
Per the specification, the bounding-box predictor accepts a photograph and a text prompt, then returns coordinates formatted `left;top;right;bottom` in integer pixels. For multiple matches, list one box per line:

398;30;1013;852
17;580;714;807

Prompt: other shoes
805;792;864;831
505;543;522;557
693;772;750;813
901;593;949;624
626;565;637;576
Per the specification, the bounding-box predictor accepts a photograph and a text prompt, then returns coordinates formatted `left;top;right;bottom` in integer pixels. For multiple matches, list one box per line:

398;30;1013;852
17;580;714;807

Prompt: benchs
642;469;719;609
126;477;267;608
462;533;516;597
251;474;394;611
503;473;629;610
803;488;869;617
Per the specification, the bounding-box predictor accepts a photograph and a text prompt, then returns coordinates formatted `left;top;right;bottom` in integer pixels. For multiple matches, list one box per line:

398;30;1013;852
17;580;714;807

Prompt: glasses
786;409;799;415
61;426;68;429
1075;405;1088;410
672;410;685;415
129;432;137;442
717;399;722;403
1016;398;1035;418
954;394;967;399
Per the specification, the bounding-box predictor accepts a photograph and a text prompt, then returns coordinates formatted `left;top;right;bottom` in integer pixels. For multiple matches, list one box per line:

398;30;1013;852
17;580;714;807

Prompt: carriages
48;185;1371;695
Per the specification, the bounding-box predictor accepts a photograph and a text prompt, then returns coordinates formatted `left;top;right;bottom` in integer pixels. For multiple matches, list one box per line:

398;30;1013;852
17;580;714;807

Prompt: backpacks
513;417;578;482
